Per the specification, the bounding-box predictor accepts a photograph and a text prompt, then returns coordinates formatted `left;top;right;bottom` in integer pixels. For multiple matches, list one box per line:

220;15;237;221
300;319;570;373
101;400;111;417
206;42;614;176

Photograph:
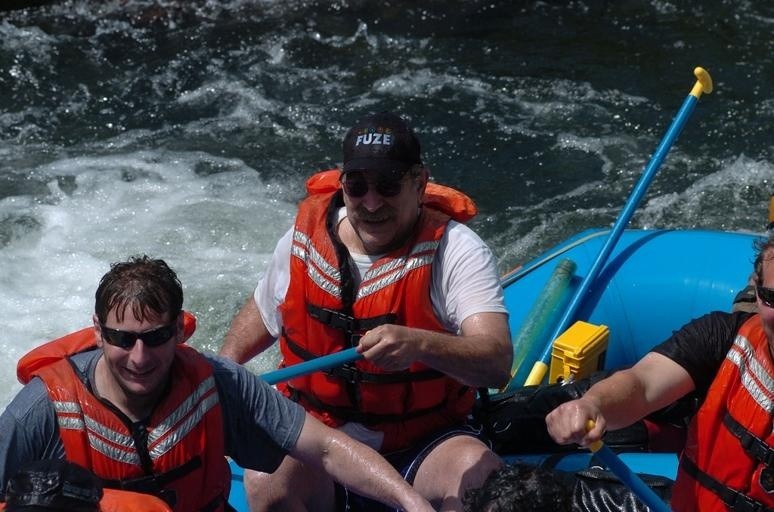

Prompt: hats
337;110;422;184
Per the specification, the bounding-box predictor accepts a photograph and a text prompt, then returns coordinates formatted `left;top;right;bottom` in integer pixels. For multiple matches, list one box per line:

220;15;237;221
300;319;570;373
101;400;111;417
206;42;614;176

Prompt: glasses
340;166;412;199
97;317;175;349
757;274;773;309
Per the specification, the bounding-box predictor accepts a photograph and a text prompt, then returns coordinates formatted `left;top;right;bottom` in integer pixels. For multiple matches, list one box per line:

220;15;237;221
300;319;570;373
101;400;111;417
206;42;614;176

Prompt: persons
0;253;437;512
216;113;513;511
542;239;773;512
